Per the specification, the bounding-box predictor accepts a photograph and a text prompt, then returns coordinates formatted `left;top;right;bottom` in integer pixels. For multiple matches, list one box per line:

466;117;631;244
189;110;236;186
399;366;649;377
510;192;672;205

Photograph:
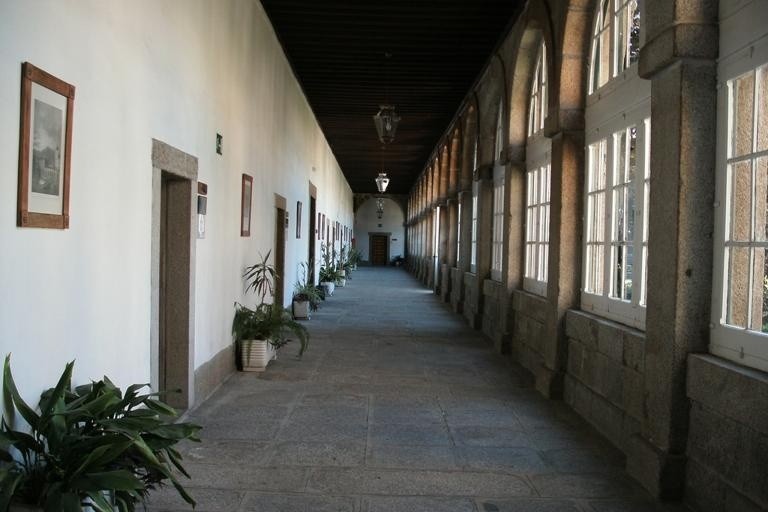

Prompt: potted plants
333;245;355;287
230;248;310;372
0;350;202;512
319;239;339;297
292;259;321;320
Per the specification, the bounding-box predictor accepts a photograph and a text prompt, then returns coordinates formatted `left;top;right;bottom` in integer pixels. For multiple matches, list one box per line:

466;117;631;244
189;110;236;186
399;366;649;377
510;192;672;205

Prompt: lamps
374;146;390;194
376;194;384;213
372;48;404;145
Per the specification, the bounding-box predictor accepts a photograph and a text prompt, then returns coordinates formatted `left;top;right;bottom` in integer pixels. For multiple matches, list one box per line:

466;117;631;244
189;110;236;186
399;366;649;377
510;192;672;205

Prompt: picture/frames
297;201;353;242
17;61;76;230
240;173;253;236
